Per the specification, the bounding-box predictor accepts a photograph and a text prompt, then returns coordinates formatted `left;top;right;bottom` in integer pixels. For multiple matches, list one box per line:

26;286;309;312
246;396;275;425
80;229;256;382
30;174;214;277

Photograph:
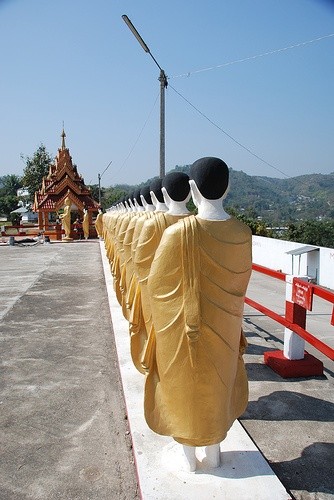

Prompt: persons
81;208;89;239
95;172;197;375
141;156;252;471
59;197;71;238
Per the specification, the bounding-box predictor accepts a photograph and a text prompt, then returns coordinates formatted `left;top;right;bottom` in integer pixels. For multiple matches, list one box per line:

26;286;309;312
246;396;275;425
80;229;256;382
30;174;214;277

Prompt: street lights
121;12;169;177
